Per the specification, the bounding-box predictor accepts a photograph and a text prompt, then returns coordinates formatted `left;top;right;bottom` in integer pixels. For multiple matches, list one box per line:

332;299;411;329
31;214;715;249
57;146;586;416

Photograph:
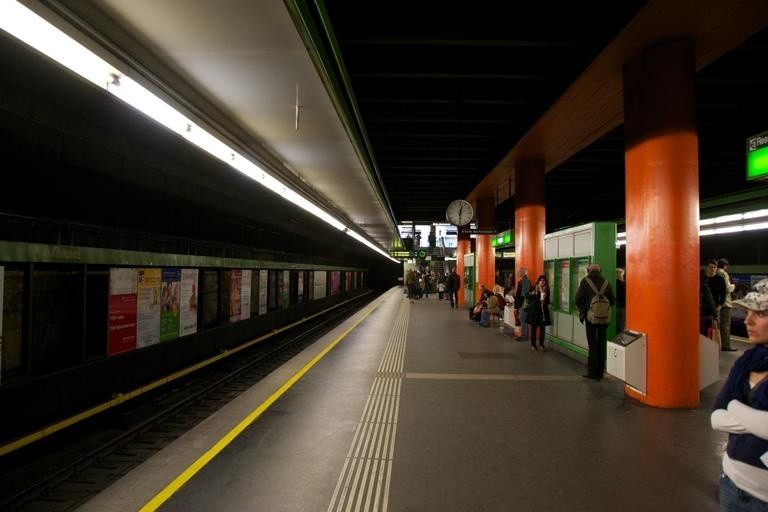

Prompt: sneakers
580;370;604;382
721;346;738;351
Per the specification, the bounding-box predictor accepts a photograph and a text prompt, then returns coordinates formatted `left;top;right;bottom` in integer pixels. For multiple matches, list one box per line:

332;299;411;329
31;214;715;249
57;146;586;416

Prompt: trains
611;223;768;320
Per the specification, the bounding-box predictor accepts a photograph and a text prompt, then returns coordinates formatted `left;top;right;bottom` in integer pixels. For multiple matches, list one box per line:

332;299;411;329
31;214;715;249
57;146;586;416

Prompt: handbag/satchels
522;296;530;312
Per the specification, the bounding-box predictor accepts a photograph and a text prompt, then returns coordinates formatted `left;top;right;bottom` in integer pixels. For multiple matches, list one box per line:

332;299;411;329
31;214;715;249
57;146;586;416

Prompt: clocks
446;199;474;227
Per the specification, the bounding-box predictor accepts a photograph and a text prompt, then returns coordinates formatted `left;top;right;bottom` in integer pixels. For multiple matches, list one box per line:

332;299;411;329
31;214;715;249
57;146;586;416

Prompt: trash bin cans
504;294;516;328
607;329;647;397
700;333;719;392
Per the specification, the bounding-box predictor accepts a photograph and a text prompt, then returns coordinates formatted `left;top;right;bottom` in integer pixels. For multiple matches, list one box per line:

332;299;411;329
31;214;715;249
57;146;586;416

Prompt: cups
541;292;545;300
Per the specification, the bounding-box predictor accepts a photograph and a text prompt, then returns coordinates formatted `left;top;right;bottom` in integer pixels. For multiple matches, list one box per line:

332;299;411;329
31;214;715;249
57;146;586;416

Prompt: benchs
487;298;510;326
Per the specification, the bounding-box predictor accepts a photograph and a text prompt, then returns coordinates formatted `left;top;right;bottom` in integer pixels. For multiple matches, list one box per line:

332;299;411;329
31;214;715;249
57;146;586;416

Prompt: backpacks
583;276;612;325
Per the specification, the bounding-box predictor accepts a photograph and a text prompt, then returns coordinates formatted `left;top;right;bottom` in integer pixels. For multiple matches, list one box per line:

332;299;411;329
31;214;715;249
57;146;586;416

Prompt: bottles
506;299;510;306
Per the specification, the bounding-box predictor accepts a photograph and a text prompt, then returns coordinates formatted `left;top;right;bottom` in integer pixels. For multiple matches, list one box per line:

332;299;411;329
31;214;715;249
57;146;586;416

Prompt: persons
428;232;437;250
472;268;515;328
404;267;460;307
160;282;181;311
700;257;739;351
514;268;533;340
231;283;240;304
709;277;768;511
525;275;552;354
416;234;421;247
575;263;616;382
618;246;629;307
278;281;291;308
407;233;411;238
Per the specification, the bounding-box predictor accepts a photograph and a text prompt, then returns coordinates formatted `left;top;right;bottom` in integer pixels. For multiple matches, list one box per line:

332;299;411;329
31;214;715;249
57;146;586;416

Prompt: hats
729;277;768;312
